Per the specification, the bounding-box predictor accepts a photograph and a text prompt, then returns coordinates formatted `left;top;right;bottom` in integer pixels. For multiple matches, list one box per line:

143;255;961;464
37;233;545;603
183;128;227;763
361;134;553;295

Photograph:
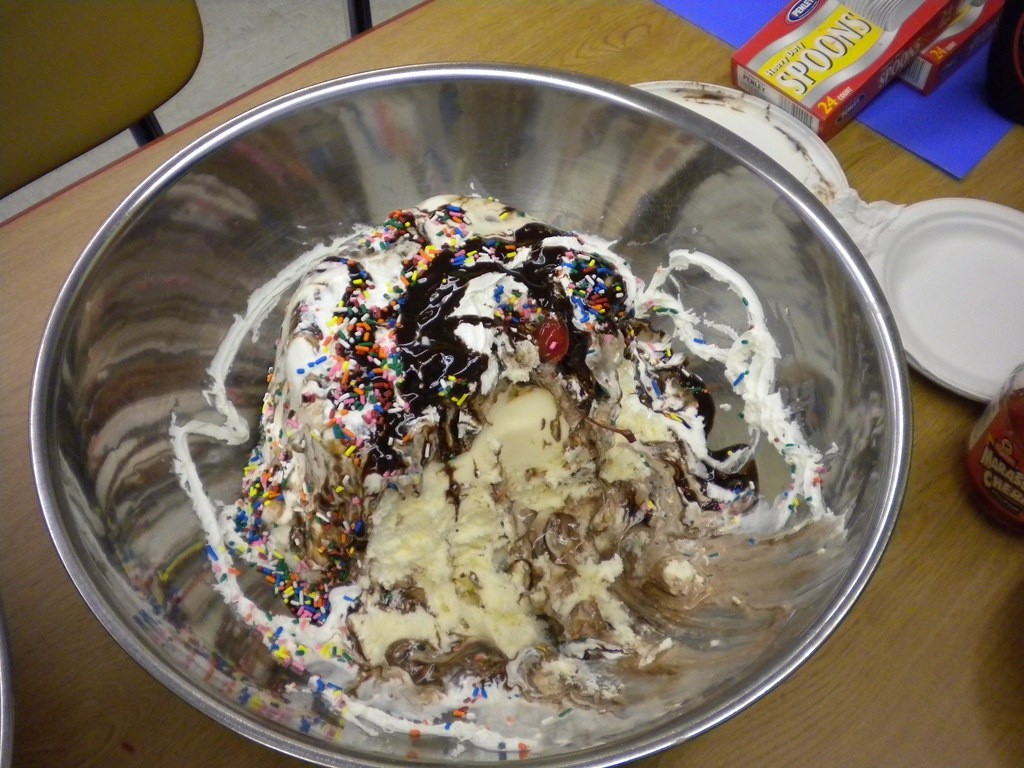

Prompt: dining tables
0;0;1024;768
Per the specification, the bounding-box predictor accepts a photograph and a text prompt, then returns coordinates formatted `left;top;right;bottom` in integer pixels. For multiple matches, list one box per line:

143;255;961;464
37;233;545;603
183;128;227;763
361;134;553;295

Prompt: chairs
0;0;205;200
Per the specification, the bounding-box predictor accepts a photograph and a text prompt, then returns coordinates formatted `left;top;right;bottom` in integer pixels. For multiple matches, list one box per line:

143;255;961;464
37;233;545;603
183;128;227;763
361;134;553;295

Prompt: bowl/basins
38;70;909;768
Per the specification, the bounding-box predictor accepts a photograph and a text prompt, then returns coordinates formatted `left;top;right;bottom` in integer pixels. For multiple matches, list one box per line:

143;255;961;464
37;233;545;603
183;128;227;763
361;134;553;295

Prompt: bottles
966;364;1024;533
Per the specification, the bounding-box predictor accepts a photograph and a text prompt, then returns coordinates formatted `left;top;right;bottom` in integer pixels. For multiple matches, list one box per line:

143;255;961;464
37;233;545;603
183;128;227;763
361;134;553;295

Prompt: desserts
248;209;752;680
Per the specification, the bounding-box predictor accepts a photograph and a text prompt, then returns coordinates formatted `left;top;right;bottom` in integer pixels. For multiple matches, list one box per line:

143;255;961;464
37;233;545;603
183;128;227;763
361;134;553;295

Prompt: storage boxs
894;0;1007;97
729;0;960;141
